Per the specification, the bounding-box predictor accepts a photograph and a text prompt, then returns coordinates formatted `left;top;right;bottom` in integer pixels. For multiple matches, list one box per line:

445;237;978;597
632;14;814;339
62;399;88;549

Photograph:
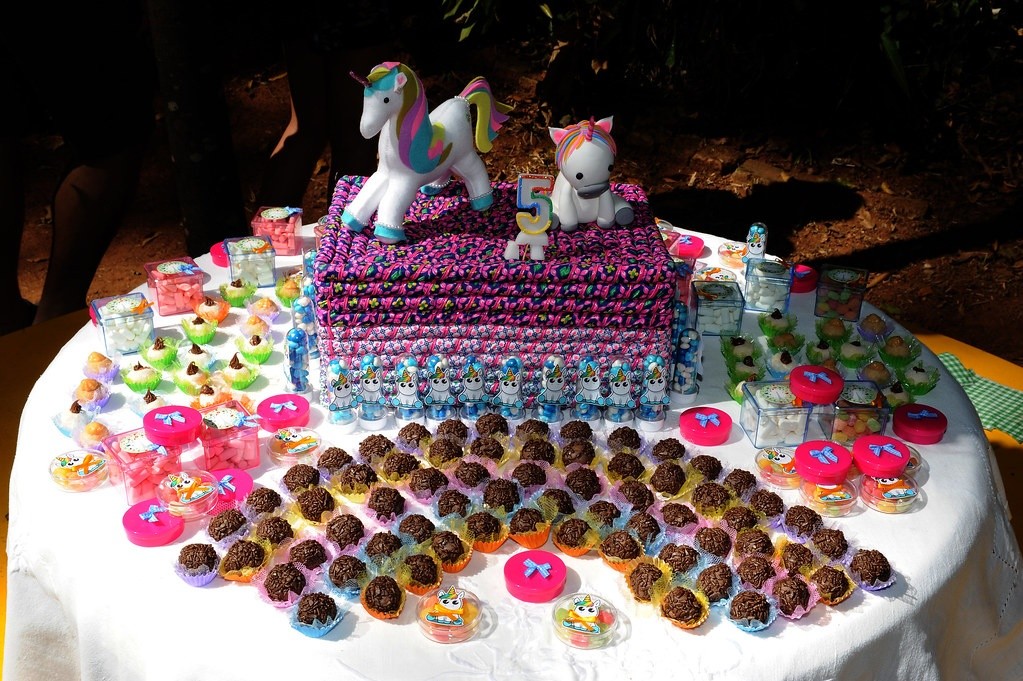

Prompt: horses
339;61;513;244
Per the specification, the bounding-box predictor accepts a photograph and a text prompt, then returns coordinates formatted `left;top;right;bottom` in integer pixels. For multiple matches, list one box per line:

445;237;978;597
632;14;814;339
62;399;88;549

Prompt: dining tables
3;188;1023;681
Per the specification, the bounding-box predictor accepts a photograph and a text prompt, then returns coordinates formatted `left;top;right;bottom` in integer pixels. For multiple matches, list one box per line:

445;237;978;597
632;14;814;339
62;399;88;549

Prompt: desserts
54;204;949;650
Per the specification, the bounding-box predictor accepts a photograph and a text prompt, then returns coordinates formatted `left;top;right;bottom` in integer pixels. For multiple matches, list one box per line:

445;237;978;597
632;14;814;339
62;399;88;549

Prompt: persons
0;0;393;326
